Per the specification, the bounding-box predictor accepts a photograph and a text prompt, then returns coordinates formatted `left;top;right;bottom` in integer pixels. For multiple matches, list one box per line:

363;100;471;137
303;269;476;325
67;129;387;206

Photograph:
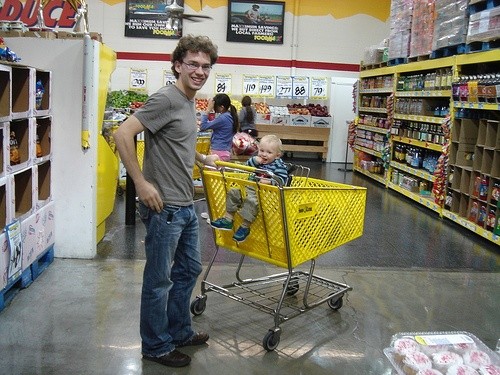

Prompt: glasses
182;61;212;70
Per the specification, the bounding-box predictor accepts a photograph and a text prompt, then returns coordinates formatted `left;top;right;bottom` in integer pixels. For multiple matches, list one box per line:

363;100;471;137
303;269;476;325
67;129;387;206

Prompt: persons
112;36;224;367
209;134;288;242
236;95;257;137
200;93;238;224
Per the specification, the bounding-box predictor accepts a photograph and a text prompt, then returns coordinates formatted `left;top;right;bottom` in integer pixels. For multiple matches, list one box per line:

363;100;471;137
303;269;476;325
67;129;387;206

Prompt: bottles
395;70;453;90
393;119;449;146
357;113;389;130
451;73;500;103
394;96;451;118
360;94;388;109
390;168;434;196
392;142;440;174
359;75;394;89
444;167;500;233
361;159;385;174
355;130;386;153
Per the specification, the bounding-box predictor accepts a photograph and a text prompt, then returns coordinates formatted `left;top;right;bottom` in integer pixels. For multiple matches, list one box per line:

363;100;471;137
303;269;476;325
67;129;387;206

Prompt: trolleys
193;129;214;180
190;158;367;352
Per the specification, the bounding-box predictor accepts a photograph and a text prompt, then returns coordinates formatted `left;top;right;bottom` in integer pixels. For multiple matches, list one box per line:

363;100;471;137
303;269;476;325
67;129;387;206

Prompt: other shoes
201;212;211;223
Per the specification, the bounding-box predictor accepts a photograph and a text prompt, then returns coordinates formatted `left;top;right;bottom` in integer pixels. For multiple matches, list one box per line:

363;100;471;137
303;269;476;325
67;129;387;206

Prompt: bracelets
201;154;207;162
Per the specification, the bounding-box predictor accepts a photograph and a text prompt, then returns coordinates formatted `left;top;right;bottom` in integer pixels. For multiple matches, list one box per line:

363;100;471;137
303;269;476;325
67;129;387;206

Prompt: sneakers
232;225;250;242
210;218;233;230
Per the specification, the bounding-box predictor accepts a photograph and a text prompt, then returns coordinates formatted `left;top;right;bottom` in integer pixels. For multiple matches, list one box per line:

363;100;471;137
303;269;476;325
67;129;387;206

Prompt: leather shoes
175;331;209;345
142;349;190;367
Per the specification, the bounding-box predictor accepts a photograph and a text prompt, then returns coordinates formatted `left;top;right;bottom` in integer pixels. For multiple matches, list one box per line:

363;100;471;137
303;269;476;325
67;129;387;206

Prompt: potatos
287;103;329;117
254;102;269;114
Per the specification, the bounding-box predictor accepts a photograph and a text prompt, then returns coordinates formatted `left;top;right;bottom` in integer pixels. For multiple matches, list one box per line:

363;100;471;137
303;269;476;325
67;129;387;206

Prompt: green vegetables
106;89;149;108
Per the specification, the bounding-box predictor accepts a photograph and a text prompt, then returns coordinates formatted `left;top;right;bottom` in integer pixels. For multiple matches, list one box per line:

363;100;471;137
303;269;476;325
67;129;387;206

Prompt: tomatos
195;98;209;112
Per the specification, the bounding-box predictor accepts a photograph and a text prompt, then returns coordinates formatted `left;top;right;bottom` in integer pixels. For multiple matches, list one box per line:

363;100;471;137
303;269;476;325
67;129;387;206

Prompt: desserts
393;336;500;375
